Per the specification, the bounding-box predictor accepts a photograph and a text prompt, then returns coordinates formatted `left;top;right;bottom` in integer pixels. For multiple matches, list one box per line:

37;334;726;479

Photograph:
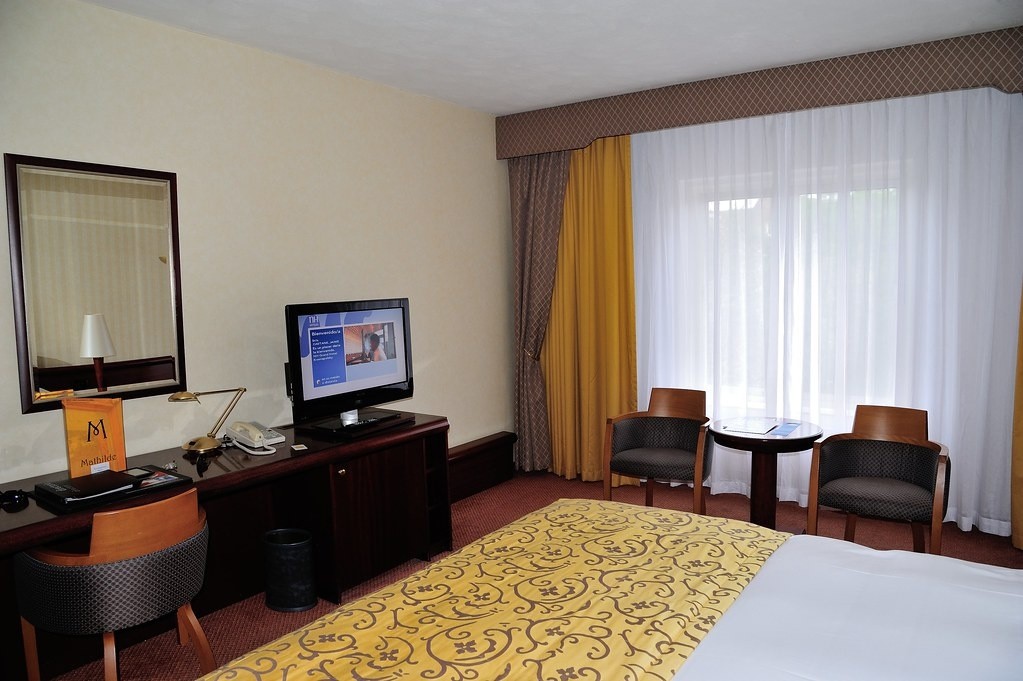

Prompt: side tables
709;416;824;535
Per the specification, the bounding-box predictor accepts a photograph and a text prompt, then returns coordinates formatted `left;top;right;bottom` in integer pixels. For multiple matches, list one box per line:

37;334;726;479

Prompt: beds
197;499;1023;681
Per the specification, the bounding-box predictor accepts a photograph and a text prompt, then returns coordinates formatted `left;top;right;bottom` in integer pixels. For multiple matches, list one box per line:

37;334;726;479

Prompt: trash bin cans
265;528;321;612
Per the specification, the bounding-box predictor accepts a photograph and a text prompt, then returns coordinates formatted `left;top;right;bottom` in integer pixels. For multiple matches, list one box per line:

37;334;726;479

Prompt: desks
0;407;453;681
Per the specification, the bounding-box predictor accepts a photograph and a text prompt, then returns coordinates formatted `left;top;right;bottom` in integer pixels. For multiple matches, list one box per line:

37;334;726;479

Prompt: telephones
225;420;285;450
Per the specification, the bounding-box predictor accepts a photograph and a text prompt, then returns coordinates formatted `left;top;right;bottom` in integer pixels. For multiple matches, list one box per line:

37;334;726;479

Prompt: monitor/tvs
285;297;413;430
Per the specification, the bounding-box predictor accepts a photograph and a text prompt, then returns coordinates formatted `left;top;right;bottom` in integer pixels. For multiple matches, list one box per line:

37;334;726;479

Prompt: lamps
79;314;116;392
168;387;246;454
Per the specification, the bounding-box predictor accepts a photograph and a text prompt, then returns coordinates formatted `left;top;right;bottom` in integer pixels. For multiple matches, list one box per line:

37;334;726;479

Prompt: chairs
807;405;952;556
604;388;713;515
12;489;216;681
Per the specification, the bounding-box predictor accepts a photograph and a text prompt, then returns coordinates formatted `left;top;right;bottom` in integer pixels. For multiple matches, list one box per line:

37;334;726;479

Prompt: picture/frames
4;153;188;415
